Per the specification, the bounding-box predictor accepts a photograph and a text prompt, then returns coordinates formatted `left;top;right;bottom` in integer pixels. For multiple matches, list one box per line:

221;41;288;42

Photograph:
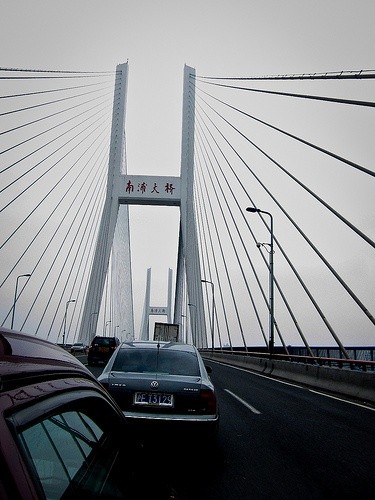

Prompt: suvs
92;335;121;363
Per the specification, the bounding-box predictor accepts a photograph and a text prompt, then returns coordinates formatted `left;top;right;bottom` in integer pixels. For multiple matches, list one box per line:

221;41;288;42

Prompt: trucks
152;322;180;342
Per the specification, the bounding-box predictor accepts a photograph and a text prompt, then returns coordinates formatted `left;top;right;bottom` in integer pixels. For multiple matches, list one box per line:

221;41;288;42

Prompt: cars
0;326;140;500
95;341;220;439
71;342;88;354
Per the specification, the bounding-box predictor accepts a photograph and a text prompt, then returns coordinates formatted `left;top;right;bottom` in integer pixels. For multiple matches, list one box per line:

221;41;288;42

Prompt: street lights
180;315;188;344
121;330;126;343
188;303;197;348
126;332;130;341
114;326;120;339
201;280;215;351
104;320;112;336
10;274;31;330
245;205;275;353
88;312;100;346
63;299;76;344
